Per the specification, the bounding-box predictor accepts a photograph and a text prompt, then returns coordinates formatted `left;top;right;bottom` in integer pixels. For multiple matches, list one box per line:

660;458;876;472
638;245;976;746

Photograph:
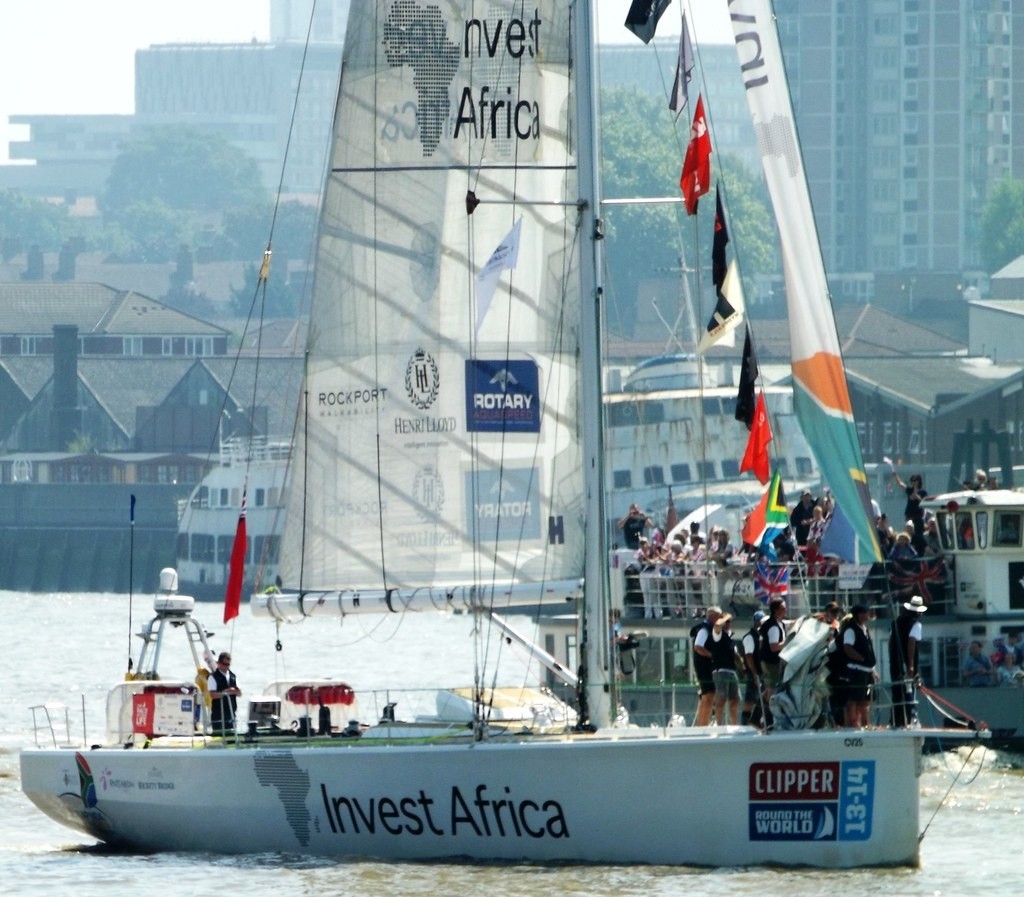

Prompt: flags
888;556;949;607
669;16;695;112
224;494;246;624
680;96;712;216
625;0;672;44
752;562;789;609
701;189;790;558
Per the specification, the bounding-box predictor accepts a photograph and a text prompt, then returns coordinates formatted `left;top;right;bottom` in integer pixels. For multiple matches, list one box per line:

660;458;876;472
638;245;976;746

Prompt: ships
177;352;826;604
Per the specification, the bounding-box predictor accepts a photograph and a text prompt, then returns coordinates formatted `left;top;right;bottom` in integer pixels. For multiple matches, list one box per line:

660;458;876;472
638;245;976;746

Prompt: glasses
220;662;230;667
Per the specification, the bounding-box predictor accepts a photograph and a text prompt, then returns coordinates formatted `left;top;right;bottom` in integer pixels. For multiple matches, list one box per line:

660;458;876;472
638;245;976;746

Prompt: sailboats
531;0;1024;756
21;0;989;866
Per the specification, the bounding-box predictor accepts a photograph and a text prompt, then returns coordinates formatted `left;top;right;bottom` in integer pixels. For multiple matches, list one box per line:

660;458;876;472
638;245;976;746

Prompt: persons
739;469;1000;603
618;506;737;568
208;652;240;744
610;609;628;680
689;595;929;729
963;631;1024;689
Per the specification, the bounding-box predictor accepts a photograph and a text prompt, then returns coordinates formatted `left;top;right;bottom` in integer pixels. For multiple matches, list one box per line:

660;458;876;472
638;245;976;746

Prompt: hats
850;604;869;614
753;611;765;621
825;602;841;612
903;596;927;613
